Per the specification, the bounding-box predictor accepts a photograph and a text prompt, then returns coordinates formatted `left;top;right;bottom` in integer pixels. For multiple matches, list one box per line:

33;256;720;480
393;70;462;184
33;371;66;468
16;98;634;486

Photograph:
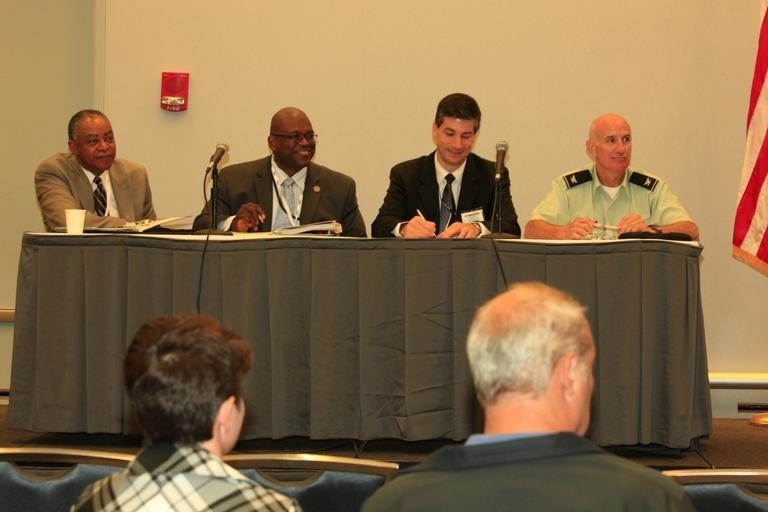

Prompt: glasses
271;131;320;143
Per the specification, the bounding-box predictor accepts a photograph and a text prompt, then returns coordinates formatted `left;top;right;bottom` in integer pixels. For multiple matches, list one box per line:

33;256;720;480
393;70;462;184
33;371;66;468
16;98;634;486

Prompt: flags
729;7;768;277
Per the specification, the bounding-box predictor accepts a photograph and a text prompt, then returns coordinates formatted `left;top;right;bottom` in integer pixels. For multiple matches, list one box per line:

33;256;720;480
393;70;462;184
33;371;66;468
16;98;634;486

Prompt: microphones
495;140;509;182
206;141;229;174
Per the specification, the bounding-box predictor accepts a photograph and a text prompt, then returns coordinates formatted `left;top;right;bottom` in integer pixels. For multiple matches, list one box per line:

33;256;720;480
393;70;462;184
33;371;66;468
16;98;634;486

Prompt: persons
361;279;693;509
519;111;699;242
368;91;522;239
64;310;304;510
193;107;366;237
32;108;161;234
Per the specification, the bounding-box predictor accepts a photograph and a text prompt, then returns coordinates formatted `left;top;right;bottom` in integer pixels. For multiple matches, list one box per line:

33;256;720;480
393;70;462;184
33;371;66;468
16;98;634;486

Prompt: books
226;219;338;238
47;212;191;234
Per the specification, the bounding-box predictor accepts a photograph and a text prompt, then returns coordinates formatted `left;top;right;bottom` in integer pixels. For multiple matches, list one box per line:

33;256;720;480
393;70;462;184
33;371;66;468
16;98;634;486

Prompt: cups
64;208;86;234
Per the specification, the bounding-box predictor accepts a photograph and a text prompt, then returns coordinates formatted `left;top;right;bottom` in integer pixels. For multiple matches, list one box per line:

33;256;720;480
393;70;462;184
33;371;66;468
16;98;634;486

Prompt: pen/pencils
258;214;264;222
595;224;617;229
416;208;436;237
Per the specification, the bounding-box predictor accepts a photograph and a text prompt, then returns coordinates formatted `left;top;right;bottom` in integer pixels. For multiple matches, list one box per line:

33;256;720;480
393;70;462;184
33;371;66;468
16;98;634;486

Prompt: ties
273;175;299;234
93;176;109;219
440;172;457;232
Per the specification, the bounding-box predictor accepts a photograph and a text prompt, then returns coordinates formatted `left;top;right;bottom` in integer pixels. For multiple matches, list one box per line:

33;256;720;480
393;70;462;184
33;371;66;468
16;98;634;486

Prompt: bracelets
473;221;483;236
650;224;663;237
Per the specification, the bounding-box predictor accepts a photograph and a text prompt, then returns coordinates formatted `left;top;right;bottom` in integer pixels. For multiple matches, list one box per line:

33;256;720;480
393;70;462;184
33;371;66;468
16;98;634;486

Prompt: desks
6;231;713;461
